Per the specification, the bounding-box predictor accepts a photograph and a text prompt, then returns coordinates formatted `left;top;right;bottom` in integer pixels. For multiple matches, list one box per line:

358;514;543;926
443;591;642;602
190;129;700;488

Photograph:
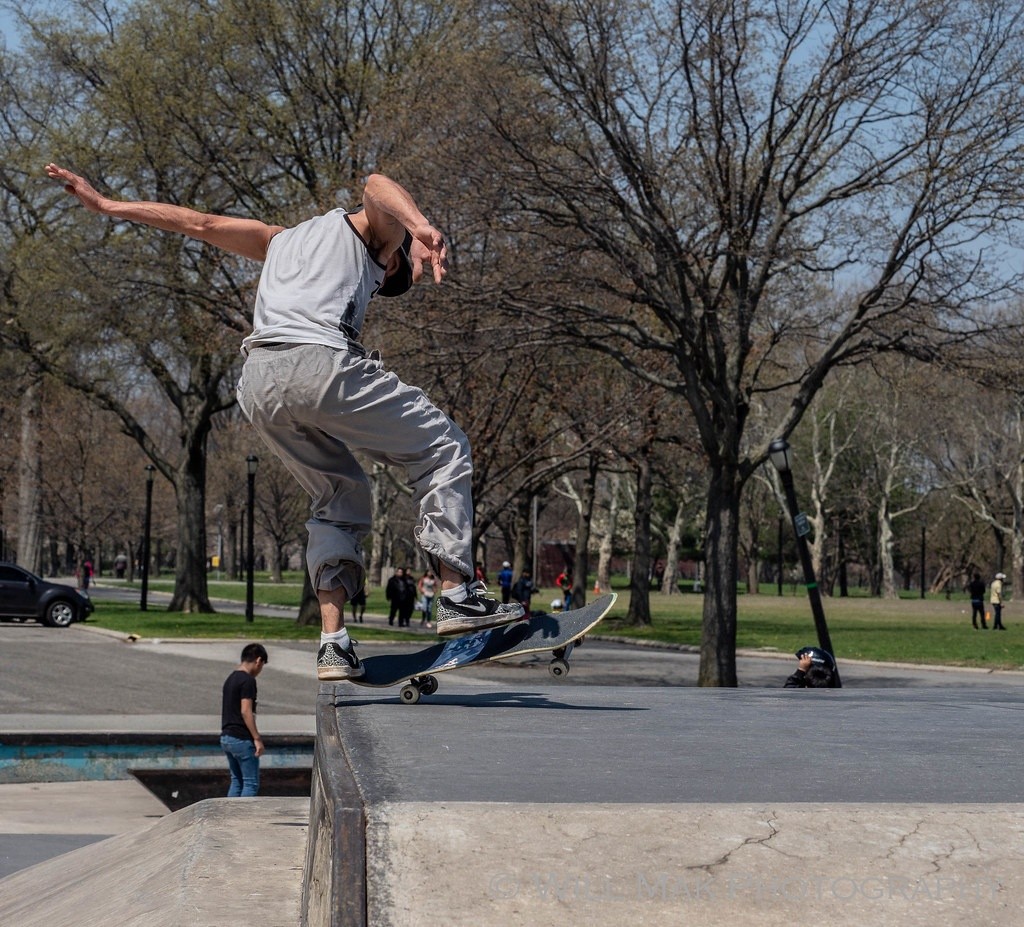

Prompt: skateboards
346;589;619;701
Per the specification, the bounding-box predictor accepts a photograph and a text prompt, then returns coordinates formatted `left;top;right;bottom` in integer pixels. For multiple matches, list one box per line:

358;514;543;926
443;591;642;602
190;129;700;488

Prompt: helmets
797;646;837;673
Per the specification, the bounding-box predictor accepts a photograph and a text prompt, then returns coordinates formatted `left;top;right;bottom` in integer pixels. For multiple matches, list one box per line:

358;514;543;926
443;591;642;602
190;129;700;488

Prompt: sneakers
436;595;526;634
317;646;365;679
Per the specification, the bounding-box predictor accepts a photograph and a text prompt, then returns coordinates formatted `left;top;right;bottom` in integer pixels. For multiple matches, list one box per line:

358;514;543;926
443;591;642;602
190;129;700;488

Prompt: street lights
244;452;260;622
768;434;842;689
139;465;158;612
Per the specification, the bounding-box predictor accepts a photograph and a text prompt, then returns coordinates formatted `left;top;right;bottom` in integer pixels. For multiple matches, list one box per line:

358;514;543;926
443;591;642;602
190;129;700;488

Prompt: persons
551;567;573;613
784;646;842;688
114;552;155;578
656;562;664;591
498;561;540;613
477;562;485;590
991;573;1006;629
84;558;95;585
386;567;438;628
45;161;525;678
968;573;988;628
221;643;269;796
350;576;368;622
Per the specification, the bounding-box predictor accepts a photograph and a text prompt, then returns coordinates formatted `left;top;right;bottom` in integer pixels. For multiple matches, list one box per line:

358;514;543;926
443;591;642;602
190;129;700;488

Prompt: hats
377;229;413;298
995;572;1007;580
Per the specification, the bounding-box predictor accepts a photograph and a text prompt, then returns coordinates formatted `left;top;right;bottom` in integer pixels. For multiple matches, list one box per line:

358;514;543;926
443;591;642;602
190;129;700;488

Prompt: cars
0;562;95;628
